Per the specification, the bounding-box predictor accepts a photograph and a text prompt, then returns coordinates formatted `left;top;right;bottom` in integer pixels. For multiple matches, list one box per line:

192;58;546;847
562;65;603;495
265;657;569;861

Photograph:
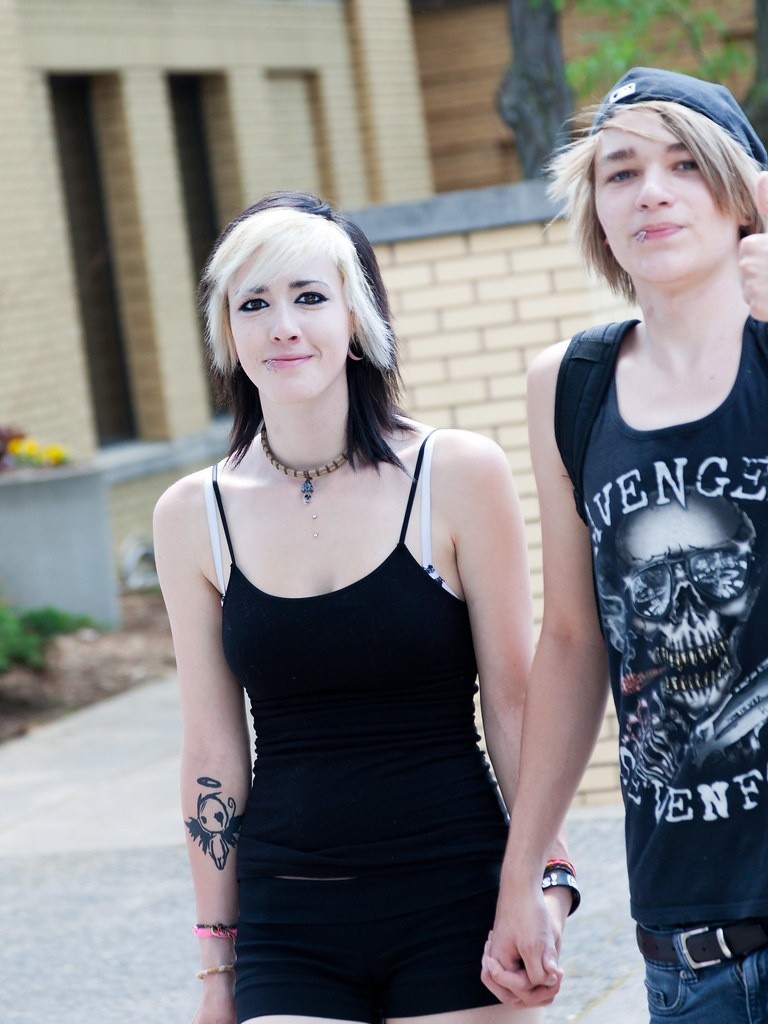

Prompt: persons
152;190;573;1024
489;67;768;1024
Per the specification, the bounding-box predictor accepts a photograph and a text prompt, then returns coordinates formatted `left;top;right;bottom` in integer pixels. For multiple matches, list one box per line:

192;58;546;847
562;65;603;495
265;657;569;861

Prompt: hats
589;66;768;169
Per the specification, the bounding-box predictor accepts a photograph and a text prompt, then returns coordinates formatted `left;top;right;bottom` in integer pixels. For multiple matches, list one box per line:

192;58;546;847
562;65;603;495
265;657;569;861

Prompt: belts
636;920;768;969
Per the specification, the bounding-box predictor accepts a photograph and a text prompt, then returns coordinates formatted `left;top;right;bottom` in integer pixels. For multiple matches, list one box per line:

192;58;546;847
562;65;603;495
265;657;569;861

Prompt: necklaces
258;422;351;505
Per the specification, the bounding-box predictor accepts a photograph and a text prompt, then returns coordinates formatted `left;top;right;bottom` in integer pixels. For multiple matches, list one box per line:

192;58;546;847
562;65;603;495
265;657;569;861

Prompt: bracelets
542;859;580;916
191;922;238;939
197;964;233;980
